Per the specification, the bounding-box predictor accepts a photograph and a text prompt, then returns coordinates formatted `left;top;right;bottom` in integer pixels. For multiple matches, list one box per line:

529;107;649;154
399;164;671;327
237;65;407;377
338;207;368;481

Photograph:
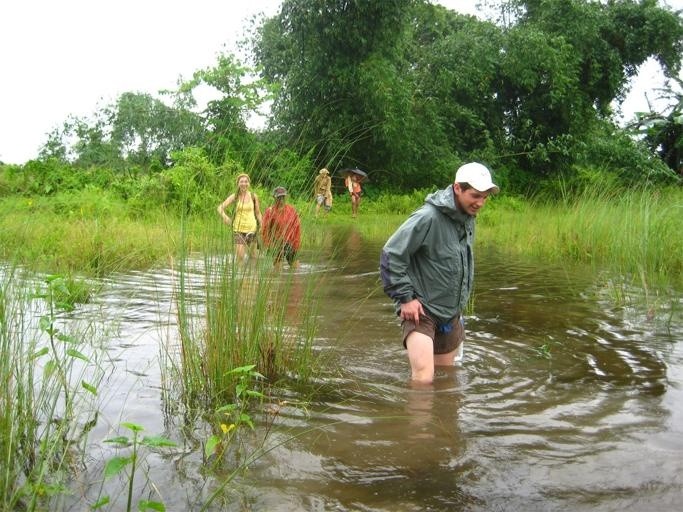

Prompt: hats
454;162;501;193
272;185;287;197
318;168;329;174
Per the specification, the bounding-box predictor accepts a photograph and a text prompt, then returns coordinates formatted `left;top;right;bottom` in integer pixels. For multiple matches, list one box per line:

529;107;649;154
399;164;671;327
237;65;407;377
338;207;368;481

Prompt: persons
378;161;501;384
344;173;365;218
313;168;333;215
215;174;263;263
260;186;302;273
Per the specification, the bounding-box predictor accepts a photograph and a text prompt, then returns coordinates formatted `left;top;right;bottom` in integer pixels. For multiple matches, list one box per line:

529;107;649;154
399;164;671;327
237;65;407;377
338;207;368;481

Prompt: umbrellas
335;166;368;179
